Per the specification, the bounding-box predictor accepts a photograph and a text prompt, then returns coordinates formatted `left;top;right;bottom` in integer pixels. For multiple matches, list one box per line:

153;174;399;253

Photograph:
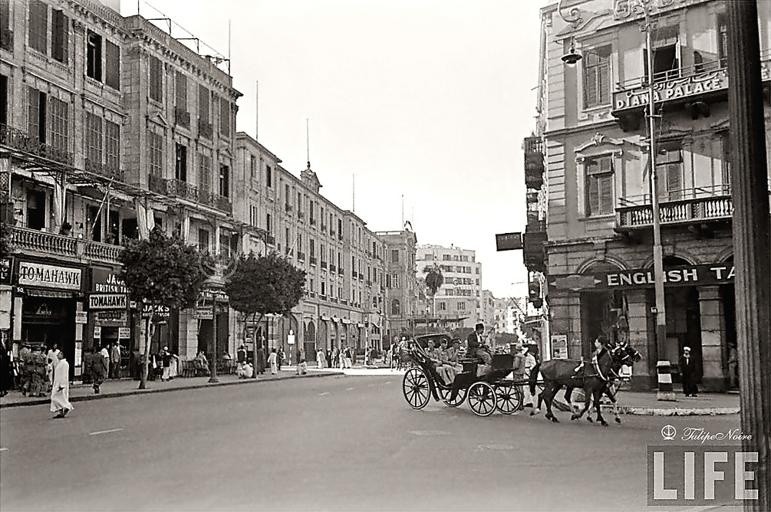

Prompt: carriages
402;323;644;428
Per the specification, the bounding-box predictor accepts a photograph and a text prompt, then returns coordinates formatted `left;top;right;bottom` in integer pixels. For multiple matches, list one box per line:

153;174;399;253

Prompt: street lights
555;0;679;402
200;289;227;384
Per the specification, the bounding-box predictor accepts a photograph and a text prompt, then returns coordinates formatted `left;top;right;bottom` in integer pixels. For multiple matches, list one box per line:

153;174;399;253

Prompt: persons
398;323;527;410
727;342;738;387
237;344;307;380
1;336;173;418
678;346;699;396
592;334;618;405
317;346;354;370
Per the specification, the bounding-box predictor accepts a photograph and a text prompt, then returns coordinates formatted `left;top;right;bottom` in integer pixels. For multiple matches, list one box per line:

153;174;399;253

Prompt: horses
527;340;643;427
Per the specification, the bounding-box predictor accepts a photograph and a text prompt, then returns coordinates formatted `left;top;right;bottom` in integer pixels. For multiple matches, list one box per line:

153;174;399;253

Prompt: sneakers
92;384;100;395
20;383;53;398
51;407;71;420
